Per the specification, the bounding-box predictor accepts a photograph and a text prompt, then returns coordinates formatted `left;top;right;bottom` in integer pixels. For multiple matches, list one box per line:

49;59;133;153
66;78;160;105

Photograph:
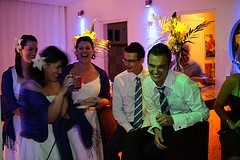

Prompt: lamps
77;10;84;17
145;0;152;7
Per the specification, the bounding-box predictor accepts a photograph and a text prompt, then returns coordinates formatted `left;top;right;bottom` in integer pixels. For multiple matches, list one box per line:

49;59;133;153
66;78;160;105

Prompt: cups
72;75;82;91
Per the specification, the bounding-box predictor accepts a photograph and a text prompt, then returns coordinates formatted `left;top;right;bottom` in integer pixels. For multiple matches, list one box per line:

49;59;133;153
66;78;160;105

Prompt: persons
214;52;240;160
105;42;150;160
17;46;77;160
0;35;42;160
170;44;208;84
60;36;110;160
143;43;210;148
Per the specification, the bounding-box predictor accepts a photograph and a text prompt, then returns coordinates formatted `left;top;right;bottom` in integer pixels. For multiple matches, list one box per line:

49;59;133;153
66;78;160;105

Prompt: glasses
122;58;141;63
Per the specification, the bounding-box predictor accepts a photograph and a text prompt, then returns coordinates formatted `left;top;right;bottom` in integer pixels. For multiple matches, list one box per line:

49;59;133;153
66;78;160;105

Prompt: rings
67;82;69;84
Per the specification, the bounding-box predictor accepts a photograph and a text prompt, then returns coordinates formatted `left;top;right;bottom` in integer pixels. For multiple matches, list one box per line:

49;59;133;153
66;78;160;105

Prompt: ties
134;78;143;130
157;86;171;115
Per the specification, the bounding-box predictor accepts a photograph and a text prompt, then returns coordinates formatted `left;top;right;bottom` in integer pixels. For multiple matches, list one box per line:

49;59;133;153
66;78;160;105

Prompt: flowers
72;29;109;52
15;36;23;53
153;13;217;72
31;53;45;70
